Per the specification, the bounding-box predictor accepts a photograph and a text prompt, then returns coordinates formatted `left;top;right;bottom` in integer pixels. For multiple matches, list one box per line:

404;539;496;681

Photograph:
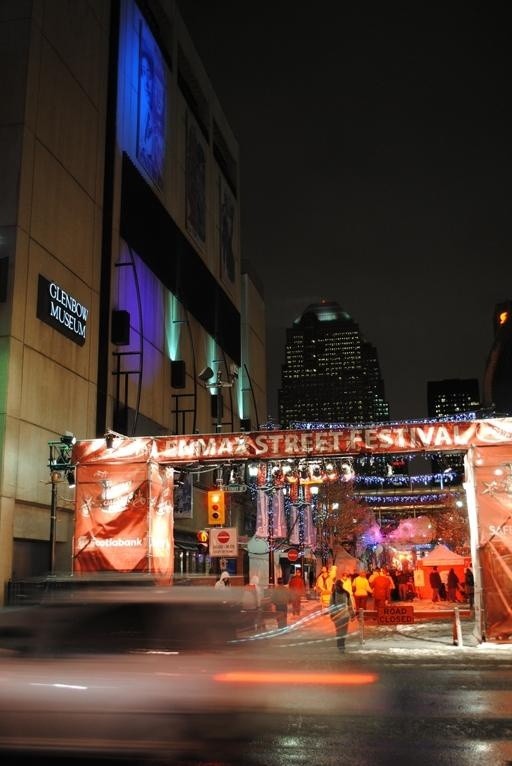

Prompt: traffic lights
196;525;209;557
207;490;225;524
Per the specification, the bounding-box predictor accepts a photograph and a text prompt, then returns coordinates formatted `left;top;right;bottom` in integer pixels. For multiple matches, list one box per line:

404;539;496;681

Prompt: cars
2;573;289;761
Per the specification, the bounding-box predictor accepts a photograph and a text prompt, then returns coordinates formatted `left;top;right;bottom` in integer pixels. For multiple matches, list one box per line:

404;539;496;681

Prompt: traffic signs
221;485;247;492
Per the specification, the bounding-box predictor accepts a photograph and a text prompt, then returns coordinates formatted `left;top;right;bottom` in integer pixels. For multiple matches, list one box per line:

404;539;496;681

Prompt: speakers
240;418;251;431
171;360;185;389
211;395;223;418
111;307;130;345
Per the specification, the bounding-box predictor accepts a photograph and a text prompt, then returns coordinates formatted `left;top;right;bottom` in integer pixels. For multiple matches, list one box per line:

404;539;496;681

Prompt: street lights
243;459;355;597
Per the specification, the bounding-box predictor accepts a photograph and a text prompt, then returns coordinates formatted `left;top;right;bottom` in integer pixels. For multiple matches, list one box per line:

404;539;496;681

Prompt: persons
315;563;473;623
271;577;294;629
330;580;355;654
242;573;263;633
212;572;232;591
290;569;307;615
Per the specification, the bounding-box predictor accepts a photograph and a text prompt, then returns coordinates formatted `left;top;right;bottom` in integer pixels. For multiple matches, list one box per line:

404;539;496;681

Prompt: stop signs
217;531;231;544
